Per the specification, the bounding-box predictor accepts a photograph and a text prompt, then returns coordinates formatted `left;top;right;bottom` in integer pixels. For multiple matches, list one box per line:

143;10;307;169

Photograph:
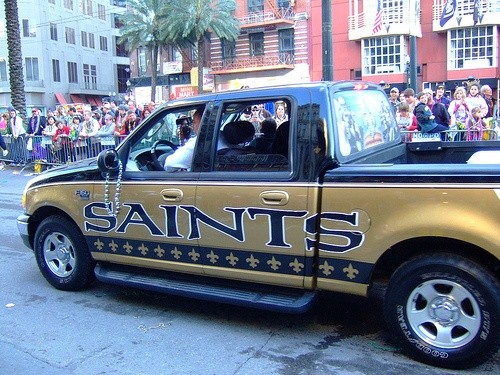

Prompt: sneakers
3;150;9;156
0;163;4;171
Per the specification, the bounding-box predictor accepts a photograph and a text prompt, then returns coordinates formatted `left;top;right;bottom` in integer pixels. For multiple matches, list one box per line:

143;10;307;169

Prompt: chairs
217;120;289;170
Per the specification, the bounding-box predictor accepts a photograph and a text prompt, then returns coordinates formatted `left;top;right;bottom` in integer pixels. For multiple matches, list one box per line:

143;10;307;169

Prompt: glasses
252;110;259;112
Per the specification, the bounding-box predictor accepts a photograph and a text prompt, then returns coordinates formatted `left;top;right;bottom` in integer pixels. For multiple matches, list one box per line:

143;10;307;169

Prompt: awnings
55;93;66;104
71;95;103;106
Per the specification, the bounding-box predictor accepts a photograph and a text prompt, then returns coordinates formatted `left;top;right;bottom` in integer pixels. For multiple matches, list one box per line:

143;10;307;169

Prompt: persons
26;105;84;171
389;84;500;141
0;110;26;166
164;109;204;171
239;102;288;150
335;95;397;156
79;99;185;158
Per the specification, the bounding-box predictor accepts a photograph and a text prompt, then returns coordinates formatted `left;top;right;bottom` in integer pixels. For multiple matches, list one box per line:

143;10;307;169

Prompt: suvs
0;105;48;120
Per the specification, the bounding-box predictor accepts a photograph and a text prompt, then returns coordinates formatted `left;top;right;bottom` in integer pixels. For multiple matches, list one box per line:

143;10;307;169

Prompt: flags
440;0;456;27
372;0;382;34
473;0;478;25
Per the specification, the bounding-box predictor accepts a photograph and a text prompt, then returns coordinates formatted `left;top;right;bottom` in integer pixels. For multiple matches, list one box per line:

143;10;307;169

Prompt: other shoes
9;162;15;166
17;163;25;167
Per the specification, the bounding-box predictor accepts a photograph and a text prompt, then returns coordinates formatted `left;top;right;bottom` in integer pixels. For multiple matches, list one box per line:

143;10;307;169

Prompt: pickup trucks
16;78;500;370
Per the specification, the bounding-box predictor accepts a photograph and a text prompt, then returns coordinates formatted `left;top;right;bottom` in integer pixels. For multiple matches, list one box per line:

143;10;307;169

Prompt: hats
32;108;37;112
118;106;124;109
390;87;398;93
422;88;433;95
436;84;444;88
